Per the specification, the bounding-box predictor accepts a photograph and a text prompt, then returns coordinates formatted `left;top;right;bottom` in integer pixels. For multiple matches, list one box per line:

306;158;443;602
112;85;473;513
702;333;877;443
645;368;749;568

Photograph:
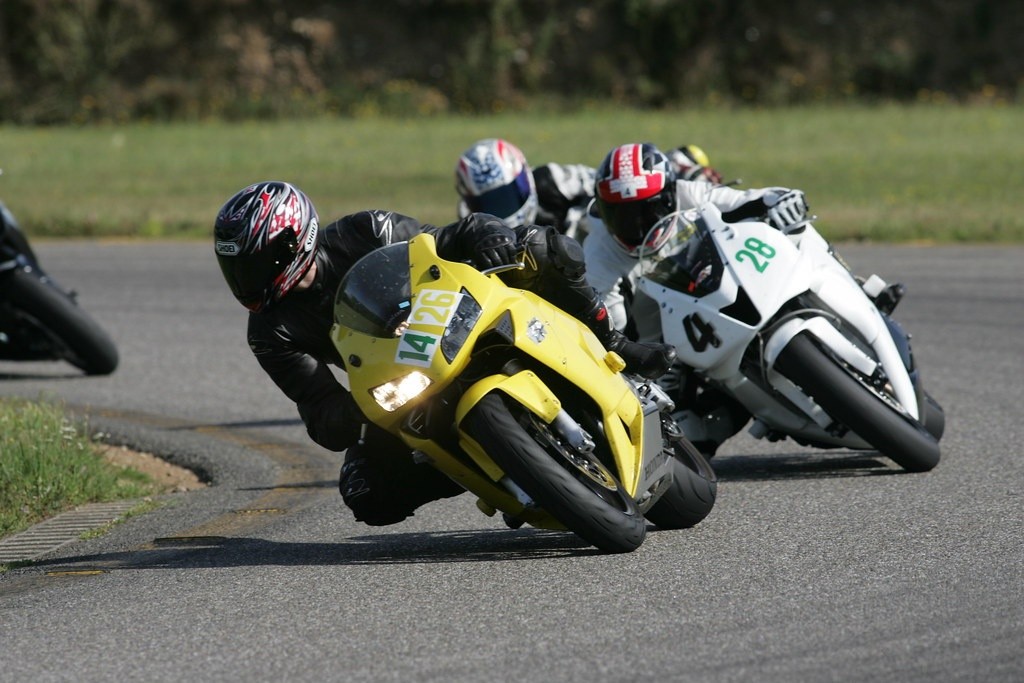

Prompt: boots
573;287;678;380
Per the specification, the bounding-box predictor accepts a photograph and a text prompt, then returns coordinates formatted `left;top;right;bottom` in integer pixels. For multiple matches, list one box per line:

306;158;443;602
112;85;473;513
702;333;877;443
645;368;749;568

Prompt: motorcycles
330;230;720;555
0;200;122;376
623;200;947;475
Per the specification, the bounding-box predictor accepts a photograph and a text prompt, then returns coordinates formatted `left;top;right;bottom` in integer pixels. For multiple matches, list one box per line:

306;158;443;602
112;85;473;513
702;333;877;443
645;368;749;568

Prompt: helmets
595;143;680;259
455;138;538;229
214;181;319;314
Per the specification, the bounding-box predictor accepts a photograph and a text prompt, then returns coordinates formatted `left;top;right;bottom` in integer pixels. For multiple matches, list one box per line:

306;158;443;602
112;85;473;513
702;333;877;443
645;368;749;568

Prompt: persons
214;180;678;526
454;138;904;459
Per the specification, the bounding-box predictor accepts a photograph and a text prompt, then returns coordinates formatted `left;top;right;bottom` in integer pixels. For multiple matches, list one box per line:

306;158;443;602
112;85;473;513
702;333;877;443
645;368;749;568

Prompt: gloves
670;159;722;186
763;189;810;231
476;233;517;268
